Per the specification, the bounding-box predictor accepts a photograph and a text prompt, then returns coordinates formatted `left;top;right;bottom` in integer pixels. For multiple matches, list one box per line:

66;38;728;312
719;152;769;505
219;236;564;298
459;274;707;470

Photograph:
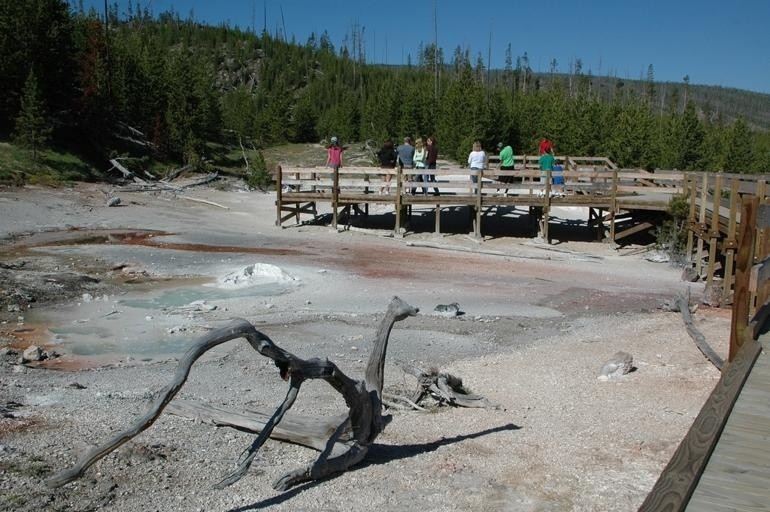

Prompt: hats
330;136;337;143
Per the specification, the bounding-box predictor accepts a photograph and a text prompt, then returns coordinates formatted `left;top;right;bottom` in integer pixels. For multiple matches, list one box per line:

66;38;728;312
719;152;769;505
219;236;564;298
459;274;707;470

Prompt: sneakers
377;189;440;196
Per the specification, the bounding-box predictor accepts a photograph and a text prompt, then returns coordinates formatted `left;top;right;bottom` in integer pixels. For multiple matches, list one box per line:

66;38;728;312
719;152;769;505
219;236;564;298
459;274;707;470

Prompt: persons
377;142;397;196
537;147;555;198
468;141;489;195
422;136;442;196
411;138;431;195
395;137;415;194
325;137;345;182
538;136;555;154
550;158;566;199
494;142;515;198
493;142;503;156
